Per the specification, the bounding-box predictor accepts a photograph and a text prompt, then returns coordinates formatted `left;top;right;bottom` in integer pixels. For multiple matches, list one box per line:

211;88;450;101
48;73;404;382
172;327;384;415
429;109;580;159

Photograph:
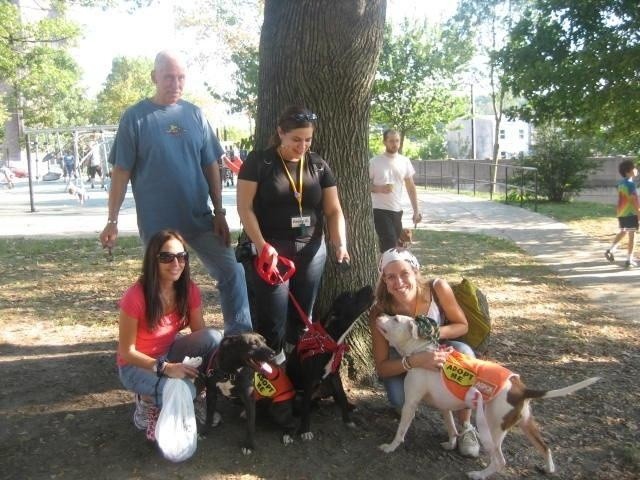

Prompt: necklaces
160;292;177;310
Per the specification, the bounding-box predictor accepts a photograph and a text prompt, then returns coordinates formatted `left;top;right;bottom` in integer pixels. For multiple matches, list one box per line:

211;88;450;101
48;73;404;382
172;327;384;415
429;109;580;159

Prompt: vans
240;149;252;161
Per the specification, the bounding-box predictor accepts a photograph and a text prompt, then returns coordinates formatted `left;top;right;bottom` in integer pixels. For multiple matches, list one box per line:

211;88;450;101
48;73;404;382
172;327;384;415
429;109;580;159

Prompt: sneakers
134;392;154;430
626;260;640;270
606;249;615;263
457;423;480;457
192;398;221;428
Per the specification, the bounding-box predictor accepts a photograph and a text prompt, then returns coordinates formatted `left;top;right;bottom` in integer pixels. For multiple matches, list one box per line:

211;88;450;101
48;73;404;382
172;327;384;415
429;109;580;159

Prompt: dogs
374;311;601;480
193;332;301;455
285;284;375;441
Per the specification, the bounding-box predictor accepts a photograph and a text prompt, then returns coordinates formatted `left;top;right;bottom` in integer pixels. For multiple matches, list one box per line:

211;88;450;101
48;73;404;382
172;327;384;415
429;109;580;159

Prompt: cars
8;167;27;178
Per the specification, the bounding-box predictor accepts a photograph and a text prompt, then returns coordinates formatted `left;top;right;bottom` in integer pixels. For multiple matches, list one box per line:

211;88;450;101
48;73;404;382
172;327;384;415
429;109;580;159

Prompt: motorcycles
0;160;15;189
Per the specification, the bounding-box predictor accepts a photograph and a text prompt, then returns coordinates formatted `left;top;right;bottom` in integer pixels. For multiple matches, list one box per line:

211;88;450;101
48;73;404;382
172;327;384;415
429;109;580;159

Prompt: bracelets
400;356;410;372
106;217;119;224
405;357;413;370
155;358;165;375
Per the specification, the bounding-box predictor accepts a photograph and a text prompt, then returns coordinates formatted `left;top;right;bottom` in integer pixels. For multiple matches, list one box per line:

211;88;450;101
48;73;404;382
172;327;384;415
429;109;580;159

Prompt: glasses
155;251;189;263
387;139;400;143
105;239;114;261
285;113;318;122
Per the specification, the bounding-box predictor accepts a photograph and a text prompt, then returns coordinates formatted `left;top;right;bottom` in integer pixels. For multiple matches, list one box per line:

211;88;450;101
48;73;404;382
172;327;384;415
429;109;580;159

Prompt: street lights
461;81;476;160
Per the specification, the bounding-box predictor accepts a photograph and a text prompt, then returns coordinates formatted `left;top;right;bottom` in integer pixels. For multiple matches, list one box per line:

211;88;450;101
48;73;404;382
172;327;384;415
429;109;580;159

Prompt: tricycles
220;141;235;187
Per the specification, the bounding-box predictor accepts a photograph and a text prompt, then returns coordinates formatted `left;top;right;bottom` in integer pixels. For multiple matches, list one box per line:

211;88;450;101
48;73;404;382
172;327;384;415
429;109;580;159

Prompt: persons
117;227;221;432
62;140;239;204
368;128;423;252
605;161;640;270
236;105;350;372
99;48;252;334
369;246;482;458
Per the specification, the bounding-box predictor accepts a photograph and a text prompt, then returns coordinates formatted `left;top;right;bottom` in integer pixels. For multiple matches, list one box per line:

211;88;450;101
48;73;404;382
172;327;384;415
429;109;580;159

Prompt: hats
377;248;418;271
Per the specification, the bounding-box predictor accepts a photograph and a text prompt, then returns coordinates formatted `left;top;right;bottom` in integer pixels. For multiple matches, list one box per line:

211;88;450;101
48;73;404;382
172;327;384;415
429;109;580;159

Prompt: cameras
234;231;256;263
